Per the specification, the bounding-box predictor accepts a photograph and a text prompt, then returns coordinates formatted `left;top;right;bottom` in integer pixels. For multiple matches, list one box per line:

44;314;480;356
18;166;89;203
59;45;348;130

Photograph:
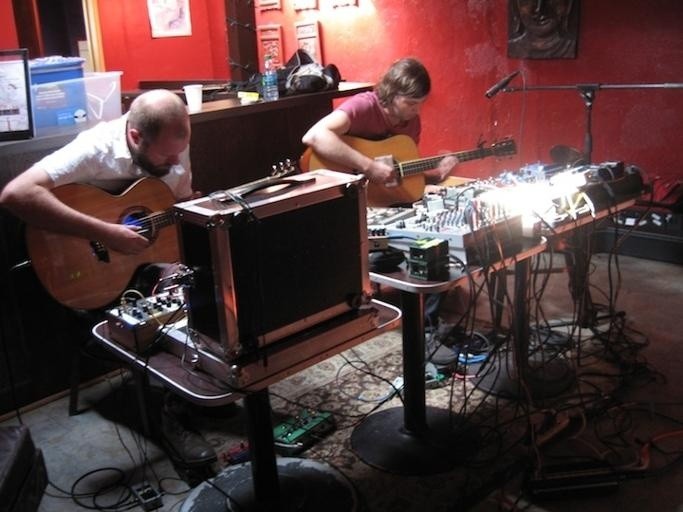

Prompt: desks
350;234;549;475
460;197;637;399
91;290;404;510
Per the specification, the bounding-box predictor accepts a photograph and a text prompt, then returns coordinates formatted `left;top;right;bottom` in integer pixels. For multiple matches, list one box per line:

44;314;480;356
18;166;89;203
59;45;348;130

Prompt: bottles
259;53;279;103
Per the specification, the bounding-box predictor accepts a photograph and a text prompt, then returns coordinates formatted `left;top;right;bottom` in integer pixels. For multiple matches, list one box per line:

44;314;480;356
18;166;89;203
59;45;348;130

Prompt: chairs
24;246;159;429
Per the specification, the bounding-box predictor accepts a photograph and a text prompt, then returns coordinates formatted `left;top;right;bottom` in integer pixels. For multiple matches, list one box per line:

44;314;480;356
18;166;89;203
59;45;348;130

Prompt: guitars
26;159;295;314
301;135;517;206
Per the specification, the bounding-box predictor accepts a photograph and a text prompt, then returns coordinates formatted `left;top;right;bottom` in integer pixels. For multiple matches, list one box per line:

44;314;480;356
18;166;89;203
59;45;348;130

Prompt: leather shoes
164;417;217;463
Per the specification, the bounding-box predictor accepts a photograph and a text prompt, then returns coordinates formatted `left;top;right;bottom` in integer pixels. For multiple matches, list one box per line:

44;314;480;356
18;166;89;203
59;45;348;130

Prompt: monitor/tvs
0;48;34;142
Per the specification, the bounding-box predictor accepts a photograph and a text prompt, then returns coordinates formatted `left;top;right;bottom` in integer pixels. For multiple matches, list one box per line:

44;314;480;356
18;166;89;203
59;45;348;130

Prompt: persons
0;89;237;468
506;0;581;59
301;59;458;387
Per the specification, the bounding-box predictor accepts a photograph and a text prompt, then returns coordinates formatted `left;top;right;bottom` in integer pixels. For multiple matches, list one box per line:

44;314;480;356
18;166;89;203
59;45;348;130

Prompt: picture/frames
294;20;324;68
293;0;319;11
256;1;281;13
330;1;358;10
257;25;285;75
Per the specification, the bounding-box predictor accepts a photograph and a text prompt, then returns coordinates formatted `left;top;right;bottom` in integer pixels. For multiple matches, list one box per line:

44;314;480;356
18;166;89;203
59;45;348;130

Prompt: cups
182;83;204;111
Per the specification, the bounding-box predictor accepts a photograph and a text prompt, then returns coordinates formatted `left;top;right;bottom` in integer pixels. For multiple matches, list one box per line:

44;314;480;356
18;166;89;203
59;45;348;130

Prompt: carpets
159;300;623;510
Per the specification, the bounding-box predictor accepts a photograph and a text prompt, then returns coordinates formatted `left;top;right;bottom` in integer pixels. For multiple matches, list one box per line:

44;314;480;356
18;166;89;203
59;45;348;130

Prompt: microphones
484;71;519;98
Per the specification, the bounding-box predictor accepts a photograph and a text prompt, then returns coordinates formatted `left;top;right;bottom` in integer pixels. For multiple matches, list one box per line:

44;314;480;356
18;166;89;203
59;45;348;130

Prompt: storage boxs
26;55;88;128
29;70;122;138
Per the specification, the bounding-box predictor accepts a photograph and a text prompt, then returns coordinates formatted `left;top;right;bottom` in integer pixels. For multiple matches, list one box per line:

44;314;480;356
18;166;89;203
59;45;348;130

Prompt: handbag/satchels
258;48;341;96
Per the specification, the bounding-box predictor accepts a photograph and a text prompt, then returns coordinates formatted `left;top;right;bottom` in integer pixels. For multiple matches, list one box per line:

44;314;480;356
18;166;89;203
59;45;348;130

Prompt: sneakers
425;334;458;363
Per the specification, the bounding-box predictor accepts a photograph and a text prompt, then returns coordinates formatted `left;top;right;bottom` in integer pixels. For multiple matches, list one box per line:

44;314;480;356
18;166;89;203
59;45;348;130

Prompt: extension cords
518;412;571;451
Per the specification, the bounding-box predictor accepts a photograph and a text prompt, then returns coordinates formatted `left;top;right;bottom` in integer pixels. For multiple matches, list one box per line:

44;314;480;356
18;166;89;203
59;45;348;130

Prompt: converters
132;481;163;512
